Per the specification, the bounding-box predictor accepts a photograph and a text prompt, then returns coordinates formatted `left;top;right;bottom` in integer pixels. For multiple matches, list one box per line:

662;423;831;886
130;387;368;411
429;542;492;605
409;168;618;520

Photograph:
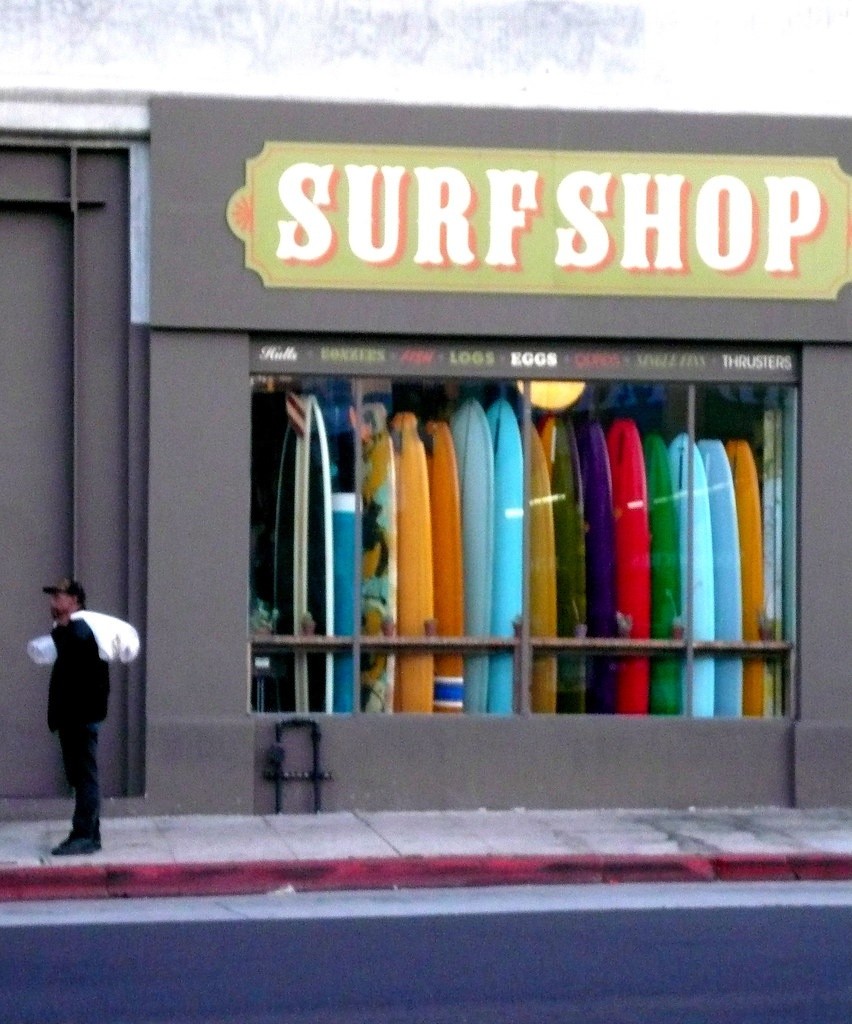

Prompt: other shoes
52;835;102;855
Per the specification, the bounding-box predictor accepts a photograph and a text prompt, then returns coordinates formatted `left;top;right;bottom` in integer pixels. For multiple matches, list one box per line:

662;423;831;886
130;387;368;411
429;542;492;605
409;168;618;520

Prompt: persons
42;578;111;856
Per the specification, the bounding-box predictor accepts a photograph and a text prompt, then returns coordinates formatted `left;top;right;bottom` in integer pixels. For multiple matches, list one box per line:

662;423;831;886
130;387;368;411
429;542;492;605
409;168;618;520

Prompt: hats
42;578;87;606
